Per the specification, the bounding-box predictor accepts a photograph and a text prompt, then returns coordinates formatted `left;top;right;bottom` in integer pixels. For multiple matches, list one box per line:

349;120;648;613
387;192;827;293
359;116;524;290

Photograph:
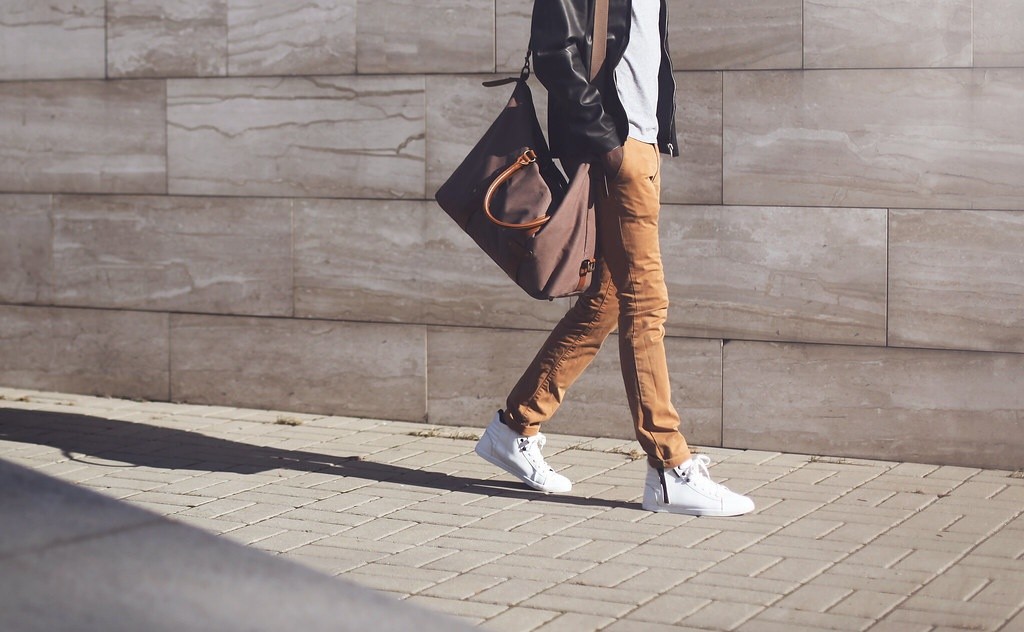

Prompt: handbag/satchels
434;57;599;302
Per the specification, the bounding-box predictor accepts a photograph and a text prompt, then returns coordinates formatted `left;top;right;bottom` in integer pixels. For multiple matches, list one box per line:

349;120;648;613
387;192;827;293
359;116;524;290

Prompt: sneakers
478;409;571;493
641;453;754;518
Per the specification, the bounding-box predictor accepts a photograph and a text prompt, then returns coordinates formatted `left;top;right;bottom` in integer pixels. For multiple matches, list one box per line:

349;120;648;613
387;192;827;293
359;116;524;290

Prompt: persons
474;0;755;518
